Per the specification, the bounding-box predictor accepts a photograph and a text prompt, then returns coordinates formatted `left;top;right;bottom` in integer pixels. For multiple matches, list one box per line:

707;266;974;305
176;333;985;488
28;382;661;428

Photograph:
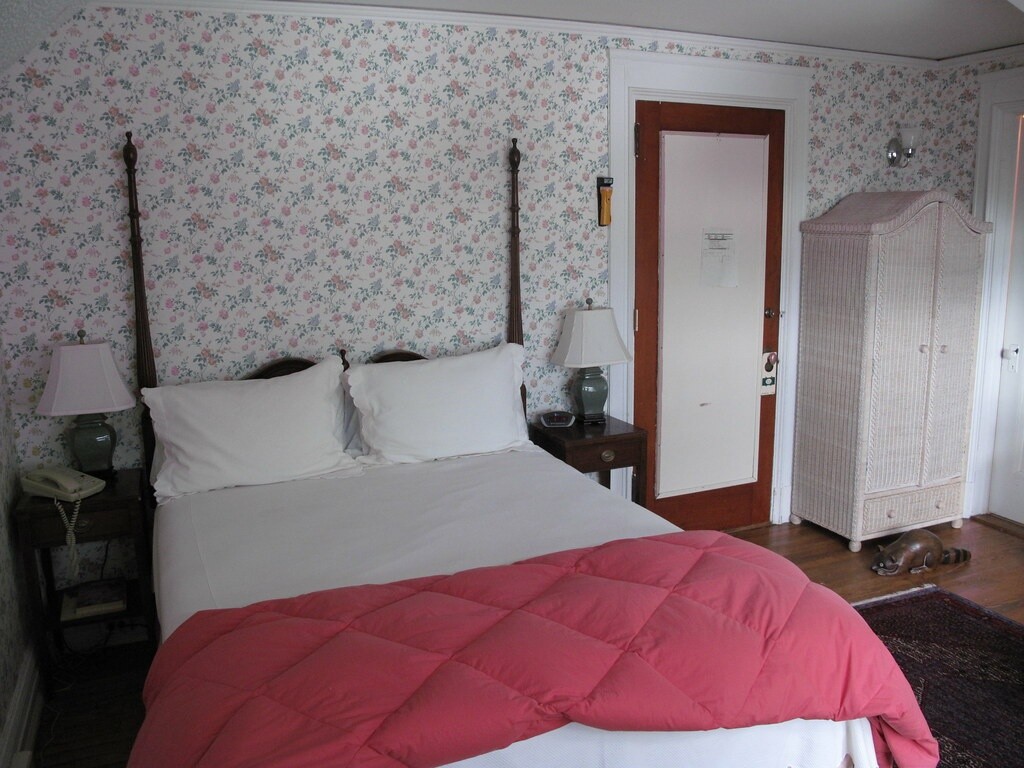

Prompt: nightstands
14;468;158;699
528;415;648;506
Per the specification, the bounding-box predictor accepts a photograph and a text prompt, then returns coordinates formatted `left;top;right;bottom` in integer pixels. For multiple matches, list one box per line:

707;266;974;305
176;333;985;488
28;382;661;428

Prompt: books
58;579;126;623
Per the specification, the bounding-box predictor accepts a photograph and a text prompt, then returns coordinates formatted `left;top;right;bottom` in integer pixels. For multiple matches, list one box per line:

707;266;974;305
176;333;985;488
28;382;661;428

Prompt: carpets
858;584;1024;768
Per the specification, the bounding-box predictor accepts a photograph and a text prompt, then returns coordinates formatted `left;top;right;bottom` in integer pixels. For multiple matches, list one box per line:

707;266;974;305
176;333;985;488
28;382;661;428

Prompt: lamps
36;331;136;484
886;125;923;168
551;299;636;425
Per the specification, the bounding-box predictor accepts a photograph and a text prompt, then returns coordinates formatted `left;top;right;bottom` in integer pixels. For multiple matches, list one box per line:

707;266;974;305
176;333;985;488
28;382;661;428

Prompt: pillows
141;341;541;502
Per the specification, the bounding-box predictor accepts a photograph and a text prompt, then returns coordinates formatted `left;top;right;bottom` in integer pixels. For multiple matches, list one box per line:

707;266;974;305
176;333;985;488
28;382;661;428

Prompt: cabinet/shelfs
791;192;995;553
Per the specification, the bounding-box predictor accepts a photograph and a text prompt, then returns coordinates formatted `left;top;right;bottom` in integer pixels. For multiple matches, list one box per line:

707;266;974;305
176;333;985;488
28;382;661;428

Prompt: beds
118;130;942;768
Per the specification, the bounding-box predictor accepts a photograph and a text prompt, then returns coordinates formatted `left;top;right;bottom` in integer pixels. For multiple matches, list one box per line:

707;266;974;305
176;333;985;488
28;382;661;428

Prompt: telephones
19;464;107;502
